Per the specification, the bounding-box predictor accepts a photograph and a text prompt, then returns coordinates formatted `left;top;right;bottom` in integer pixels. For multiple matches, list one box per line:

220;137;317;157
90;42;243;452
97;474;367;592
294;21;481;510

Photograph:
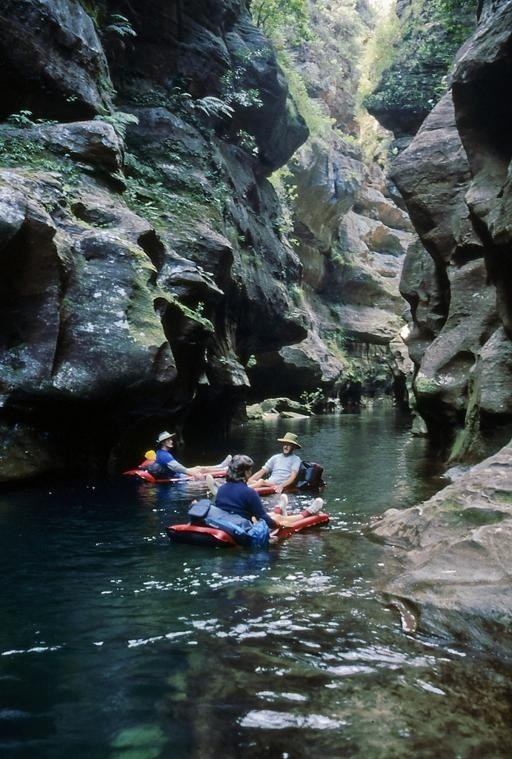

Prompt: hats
277;432;302;449
156;431;176;444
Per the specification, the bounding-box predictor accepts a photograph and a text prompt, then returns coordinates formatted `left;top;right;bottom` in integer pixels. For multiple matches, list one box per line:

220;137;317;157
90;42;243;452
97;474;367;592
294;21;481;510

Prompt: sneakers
306;497;323;516
206;475;218;495
275;494;288;516
222;455;233;471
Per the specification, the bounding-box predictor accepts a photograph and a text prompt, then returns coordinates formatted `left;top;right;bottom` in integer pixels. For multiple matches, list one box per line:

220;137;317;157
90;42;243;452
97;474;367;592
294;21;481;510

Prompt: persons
205;431;302;495
216;452;323;531
155;430;231;481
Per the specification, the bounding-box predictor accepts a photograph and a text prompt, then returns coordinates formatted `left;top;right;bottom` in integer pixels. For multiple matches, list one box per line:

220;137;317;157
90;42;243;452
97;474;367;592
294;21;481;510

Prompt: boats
122;466;230;485
252;481;323;495
166;510;329;550
